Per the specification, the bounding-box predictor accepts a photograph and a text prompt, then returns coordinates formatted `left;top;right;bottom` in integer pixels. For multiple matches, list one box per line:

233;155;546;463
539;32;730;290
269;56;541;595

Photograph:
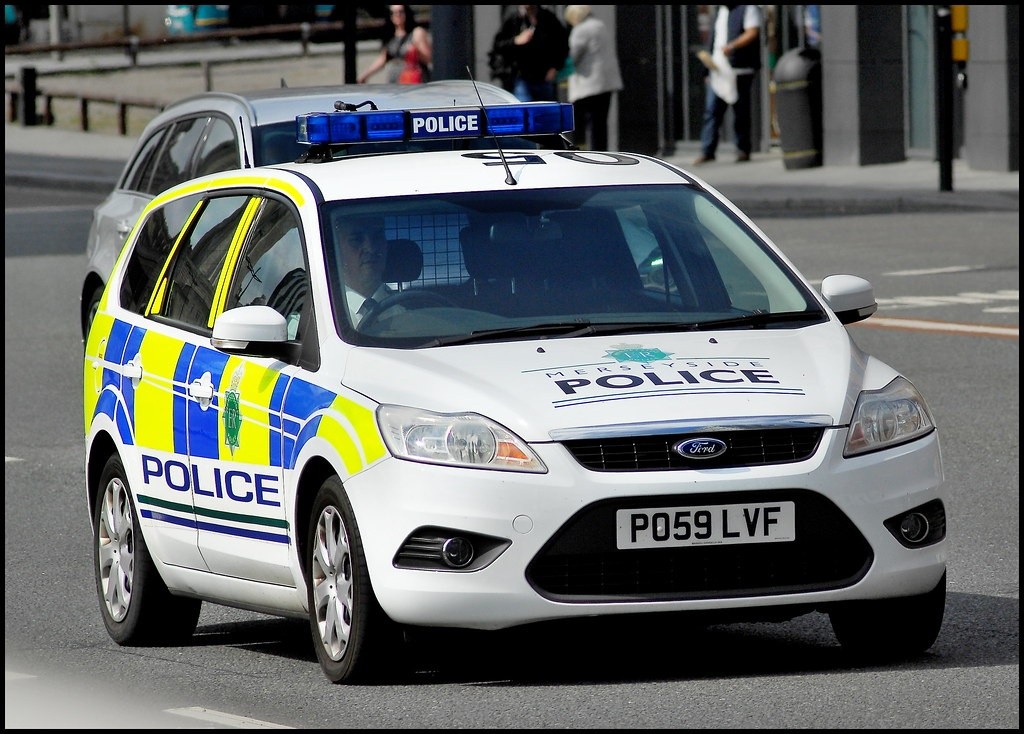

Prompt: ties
363;298;380;327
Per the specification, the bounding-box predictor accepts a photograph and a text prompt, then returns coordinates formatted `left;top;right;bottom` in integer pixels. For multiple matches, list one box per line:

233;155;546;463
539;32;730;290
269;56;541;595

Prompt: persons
357;5;431;84
287;214;417;343
487;5;624;152
694;4;822;164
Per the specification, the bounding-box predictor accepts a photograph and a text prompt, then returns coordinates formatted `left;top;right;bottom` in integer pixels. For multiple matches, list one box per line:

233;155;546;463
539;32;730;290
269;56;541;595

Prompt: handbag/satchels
397;47;425;86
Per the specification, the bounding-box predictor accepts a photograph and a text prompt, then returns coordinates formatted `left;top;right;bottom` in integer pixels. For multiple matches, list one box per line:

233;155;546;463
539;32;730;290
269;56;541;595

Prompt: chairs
382;239;422;292
536;211;653;315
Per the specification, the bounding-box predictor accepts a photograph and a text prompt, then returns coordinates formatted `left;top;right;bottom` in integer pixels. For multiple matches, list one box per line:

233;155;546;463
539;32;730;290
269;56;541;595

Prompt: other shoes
692;152;716;167
739;150;749;161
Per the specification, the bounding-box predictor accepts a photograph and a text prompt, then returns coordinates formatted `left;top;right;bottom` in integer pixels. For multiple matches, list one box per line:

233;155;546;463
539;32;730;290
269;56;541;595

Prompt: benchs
462;224;533;315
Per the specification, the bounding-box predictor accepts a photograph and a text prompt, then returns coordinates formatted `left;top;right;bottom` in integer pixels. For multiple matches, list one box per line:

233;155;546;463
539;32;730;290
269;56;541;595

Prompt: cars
83;100;954;684
78;80;678;349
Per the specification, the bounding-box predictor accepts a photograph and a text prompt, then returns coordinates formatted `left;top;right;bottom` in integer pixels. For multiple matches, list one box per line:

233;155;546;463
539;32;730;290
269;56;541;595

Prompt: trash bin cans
773;46;824;171
